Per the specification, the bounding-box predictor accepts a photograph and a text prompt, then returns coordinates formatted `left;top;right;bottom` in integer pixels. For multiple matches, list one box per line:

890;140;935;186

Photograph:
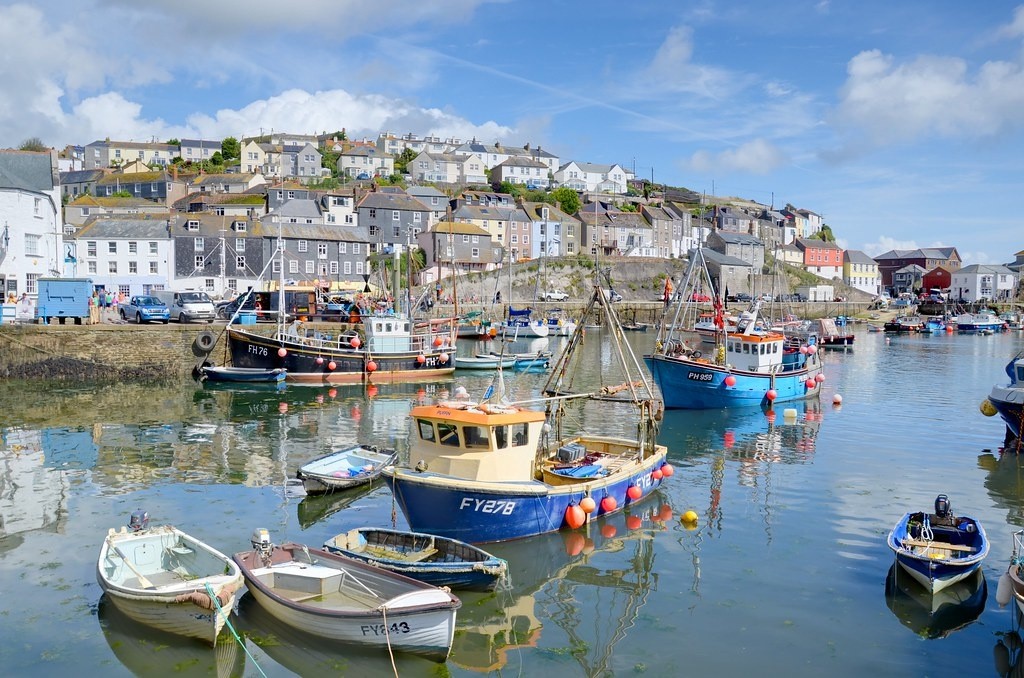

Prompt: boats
296;442;399;498
1008;528;1024;615
323;526;506;595
201;365;288;382
446;490;677;674
297;476;386;531
98;593;248;678
453;369;516;378
885;560;987;641
454;349;554;369
986;425;1024;527
1014;598;1024;629
237;590;455;678
514;365;547;374
867;300;1024;335
202;378;287;395
382;282;668;549
642;209;824;408
230;528;462;664
987;350;1024;445
656;395;820;463
225;202;460;381
886;494;989;596
96;510;245;649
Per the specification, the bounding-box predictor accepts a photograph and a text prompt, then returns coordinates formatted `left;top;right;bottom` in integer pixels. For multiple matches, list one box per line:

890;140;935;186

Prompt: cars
688;293;711;302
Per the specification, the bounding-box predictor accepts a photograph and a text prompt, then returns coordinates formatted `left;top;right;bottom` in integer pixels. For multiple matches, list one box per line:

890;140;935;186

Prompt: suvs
216;301;235;320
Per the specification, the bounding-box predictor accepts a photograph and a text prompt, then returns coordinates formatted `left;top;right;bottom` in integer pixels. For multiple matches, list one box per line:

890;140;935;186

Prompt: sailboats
458;209;578;339
620;244;855;370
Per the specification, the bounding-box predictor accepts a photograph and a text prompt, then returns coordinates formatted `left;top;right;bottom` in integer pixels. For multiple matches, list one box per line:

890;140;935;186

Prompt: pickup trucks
727;292;752;302
536;290;569;302
117;295;171;324
653;292;679;302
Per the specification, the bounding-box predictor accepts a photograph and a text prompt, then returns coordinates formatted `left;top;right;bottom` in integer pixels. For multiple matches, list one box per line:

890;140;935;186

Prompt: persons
494;290;500;303
380;293;395;302
90;289;126;324
7;293;32;325
448;294;479;304
356;294;372;322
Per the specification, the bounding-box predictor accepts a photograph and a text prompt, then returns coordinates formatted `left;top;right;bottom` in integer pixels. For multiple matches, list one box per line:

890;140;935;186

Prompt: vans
151;290;216;324
223;291;317;323
596;290;623;302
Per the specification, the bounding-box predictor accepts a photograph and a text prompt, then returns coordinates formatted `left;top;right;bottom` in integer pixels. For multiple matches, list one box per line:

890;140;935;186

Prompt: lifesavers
196;359;215;375
196;331;215;351
272;330;288;341
343;330;358;347
